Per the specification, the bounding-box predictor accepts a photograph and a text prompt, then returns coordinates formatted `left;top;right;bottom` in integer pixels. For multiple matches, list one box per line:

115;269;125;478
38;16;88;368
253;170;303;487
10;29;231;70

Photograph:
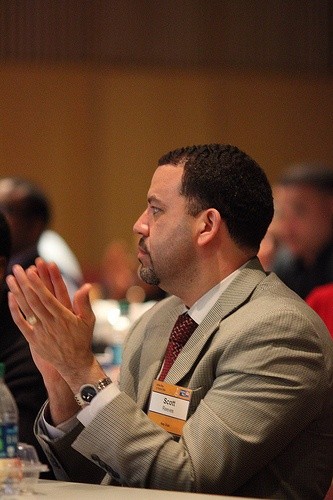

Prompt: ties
156;313;199;383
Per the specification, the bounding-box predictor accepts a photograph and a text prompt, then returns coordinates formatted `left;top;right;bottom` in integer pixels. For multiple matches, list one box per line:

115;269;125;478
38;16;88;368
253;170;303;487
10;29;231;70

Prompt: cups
18;443;39;494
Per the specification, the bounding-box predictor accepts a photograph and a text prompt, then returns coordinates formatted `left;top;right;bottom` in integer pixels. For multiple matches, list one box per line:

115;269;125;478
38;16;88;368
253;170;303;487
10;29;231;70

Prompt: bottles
0;363;18;495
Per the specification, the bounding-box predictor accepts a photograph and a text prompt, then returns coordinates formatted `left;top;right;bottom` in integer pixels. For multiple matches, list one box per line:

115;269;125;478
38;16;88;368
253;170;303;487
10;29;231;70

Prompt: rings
28;317;36;324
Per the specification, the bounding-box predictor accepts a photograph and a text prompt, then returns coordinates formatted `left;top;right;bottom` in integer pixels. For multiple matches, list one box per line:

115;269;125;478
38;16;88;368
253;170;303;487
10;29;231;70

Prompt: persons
0;177;80;480
6;144;333;500
267;157;333;296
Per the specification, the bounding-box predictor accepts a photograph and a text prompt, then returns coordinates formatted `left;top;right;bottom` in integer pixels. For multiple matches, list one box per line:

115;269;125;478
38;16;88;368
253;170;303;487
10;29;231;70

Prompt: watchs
74;376;113;406
